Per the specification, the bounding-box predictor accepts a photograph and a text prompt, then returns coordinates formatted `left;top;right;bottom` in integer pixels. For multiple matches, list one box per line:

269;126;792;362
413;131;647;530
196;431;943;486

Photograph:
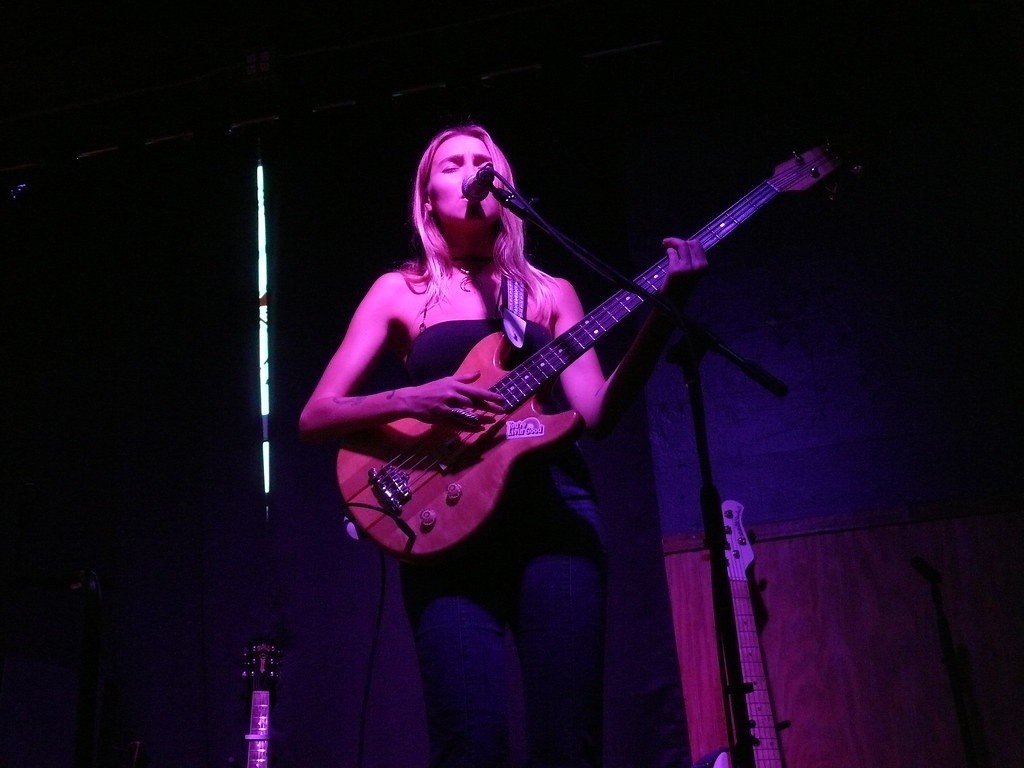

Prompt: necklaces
452;254;494;276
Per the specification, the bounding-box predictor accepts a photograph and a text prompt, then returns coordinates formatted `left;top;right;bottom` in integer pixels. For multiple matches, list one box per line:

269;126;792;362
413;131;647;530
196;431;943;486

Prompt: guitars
336;136;855;563
238;634;285;768
717;500;789;768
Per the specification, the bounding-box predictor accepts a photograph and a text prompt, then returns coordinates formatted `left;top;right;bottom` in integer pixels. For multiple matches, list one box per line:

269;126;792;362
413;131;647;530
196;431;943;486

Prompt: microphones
460;159;494;201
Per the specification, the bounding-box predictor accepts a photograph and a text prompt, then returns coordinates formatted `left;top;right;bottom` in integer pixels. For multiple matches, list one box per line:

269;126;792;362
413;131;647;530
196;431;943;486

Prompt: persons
298;126;707;768
